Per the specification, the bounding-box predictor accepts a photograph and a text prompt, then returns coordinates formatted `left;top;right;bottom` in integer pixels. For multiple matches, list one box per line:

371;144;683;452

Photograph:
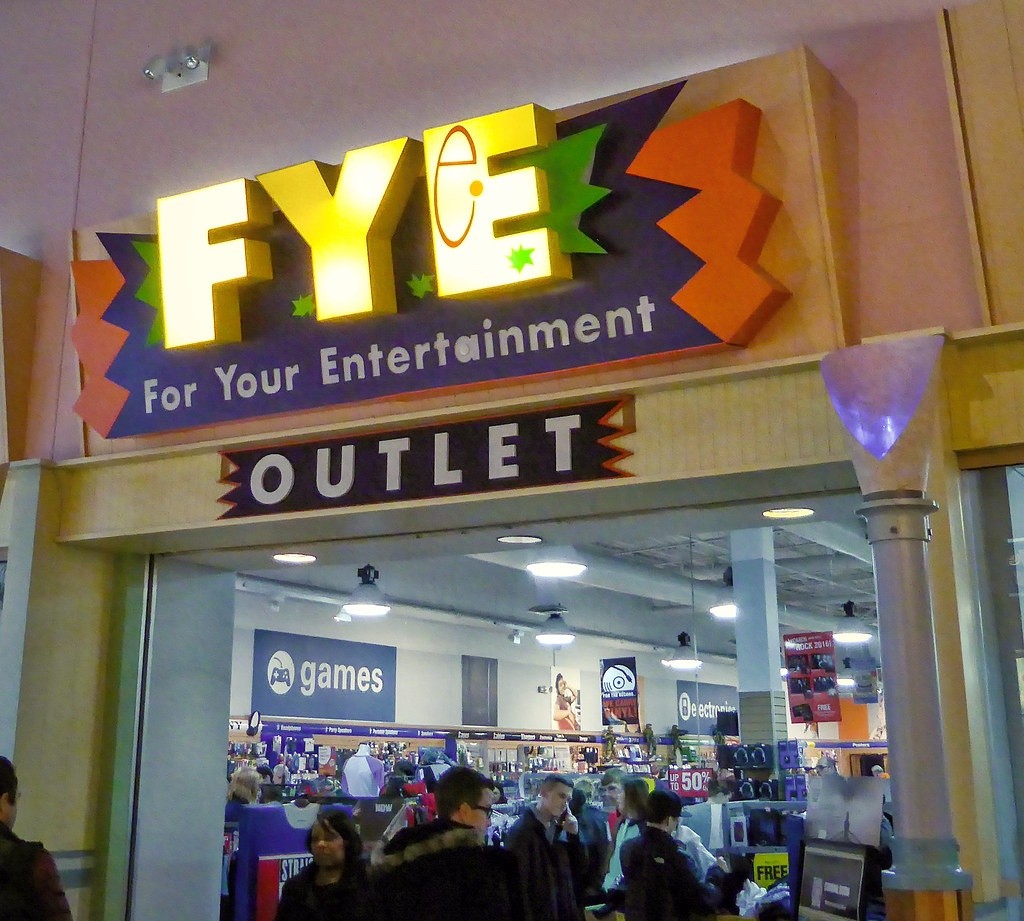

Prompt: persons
711;729;725;761
599;767;628;877
507;772;609;921
307;753;313;773
643;724;657;760
670;725;696;764
601;773;716;894
341;743;385;797
493;784;507;805
271;764;291;784
287;759;298;774
813;757;842;776
871;764;884;777
224;766;260;841
859;814;893;921
273;809;399;921
378;764;555;921
602;726;620;759
553;674;581;732
0;756;73;921
394;760;416;781
620;790;728;921
262;785;290;804
255;764;274;804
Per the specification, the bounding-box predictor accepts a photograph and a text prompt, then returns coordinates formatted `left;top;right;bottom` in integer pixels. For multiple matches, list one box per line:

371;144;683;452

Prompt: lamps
341;537;391;620
836;656;856;686
142;37;213;93
667;628;704;669
819;337;943;464
534;582;577;646
708;565;736;619
833;594;873;643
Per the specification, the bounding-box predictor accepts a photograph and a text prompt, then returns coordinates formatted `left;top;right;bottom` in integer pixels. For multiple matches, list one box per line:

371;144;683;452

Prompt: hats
648;790;692;818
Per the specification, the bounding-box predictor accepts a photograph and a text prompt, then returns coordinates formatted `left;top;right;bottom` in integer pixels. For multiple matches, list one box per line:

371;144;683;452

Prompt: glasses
476;806;492;819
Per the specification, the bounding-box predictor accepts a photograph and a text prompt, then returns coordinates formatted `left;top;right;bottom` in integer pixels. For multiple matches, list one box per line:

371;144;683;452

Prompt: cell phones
560;803;569;818
